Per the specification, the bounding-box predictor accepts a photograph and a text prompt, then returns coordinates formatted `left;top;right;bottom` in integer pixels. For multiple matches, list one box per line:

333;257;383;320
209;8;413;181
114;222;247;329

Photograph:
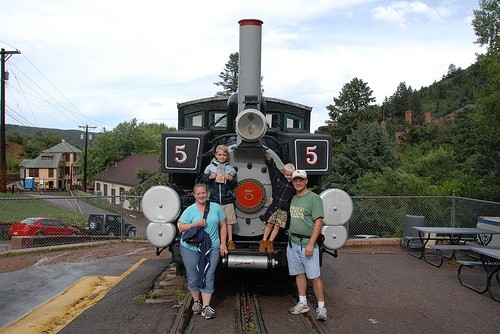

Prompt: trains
140;19;353;295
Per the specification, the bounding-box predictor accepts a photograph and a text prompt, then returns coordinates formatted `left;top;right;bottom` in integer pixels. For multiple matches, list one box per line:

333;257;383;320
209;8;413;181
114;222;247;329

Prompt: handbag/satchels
182;226;204;244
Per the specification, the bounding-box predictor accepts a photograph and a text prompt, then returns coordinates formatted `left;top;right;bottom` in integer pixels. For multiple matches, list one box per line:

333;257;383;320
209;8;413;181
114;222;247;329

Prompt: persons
259;152;295;254
202;145;238;250
269;170;328;321
178;183;228;319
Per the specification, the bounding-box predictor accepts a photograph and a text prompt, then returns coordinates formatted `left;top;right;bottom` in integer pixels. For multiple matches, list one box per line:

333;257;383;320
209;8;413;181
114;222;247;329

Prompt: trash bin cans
402;215;426;248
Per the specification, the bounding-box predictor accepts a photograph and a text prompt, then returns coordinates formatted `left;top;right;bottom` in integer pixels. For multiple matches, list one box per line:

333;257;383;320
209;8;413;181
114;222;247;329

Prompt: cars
353;234;380;239
9;217;81;236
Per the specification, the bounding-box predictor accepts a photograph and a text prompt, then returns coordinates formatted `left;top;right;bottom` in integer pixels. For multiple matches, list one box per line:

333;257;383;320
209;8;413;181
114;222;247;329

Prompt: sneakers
192;300;202;315
289;302;310;314
201;305;215;318
316;307;327;321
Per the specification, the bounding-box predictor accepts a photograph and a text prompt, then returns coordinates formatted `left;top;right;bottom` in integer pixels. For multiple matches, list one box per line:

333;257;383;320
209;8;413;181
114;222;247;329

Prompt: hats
291;170;307;179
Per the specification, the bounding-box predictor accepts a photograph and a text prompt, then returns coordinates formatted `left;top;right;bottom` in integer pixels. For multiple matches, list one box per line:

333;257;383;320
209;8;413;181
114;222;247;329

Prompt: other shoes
259;240;267;252
267;240;274;253
227;240;236;250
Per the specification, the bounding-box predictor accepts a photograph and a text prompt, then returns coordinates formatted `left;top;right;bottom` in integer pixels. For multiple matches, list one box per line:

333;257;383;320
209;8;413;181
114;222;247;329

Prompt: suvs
88;212;136;239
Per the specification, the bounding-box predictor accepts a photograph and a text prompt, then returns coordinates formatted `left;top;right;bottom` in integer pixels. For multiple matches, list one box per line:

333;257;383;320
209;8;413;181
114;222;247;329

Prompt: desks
409;227;500;260
470;247;500;298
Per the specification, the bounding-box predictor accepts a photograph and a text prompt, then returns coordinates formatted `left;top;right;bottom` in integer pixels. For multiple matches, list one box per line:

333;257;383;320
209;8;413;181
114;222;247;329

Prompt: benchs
404;236;500;294
